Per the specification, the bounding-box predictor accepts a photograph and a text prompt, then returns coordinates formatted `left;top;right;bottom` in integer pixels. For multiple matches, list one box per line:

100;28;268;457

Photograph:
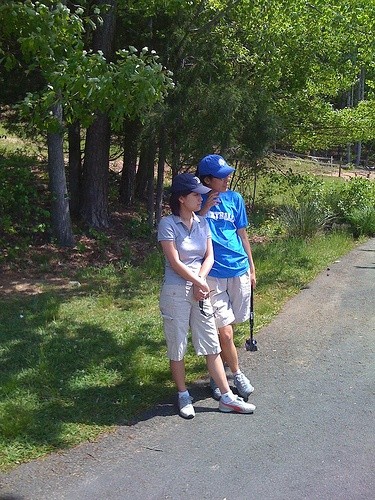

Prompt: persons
158;173;256;419
194;155;257;400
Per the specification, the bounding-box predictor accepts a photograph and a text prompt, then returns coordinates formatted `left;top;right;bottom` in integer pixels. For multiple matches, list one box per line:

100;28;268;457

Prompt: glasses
199;290;218;317
220;175;230;181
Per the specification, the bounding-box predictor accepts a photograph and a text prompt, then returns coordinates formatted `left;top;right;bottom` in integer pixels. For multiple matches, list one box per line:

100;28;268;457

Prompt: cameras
245;338;257;351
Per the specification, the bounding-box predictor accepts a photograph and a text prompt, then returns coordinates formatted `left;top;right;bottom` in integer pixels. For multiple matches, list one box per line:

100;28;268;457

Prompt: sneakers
178;395;195;419
210;376;222;401
233;373;254;398
219;394;256;413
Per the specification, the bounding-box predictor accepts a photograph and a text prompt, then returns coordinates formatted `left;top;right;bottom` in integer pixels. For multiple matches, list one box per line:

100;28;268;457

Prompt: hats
171;173;212;195
198;154;236;179
